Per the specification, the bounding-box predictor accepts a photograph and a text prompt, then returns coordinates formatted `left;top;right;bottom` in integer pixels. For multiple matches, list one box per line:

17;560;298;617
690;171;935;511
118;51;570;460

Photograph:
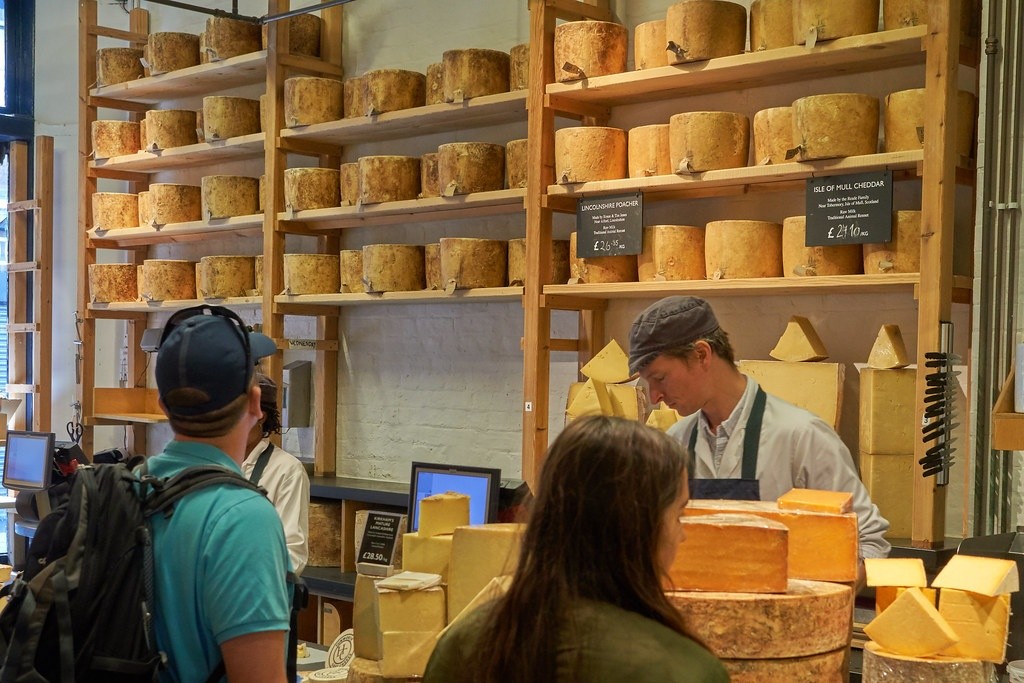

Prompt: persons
422;415;732;683
242;370;309;682
627;293;891;597
130;304;291;683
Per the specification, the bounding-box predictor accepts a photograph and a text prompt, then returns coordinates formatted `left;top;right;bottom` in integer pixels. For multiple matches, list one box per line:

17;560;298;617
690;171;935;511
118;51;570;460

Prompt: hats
628;294;719;377
155;314;277;416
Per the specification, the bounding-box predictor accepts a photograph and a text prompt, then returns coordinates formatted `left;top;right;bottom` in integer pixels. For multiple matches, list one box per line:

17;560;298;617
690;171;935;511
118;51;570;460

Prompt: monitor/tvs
2;431;56;491
406;461;501;535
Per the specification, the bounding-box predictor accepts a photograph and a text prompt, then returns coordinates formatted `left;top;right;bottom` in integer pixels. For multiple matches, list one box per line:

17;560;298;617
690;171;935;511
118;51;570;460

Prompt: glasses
159;303;253;393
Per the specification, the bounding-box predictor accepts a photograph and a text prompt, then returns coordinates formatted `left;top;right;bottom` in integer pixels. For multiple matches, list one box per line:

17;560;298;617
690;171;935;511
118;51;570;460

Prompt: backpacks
0;442;310;683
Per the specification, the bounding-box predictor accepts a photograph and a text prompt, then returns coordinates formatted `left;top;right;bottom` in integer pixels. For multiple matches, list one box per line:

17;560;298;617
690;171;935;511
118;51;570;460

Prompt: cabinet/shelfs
72;0;1024;576
